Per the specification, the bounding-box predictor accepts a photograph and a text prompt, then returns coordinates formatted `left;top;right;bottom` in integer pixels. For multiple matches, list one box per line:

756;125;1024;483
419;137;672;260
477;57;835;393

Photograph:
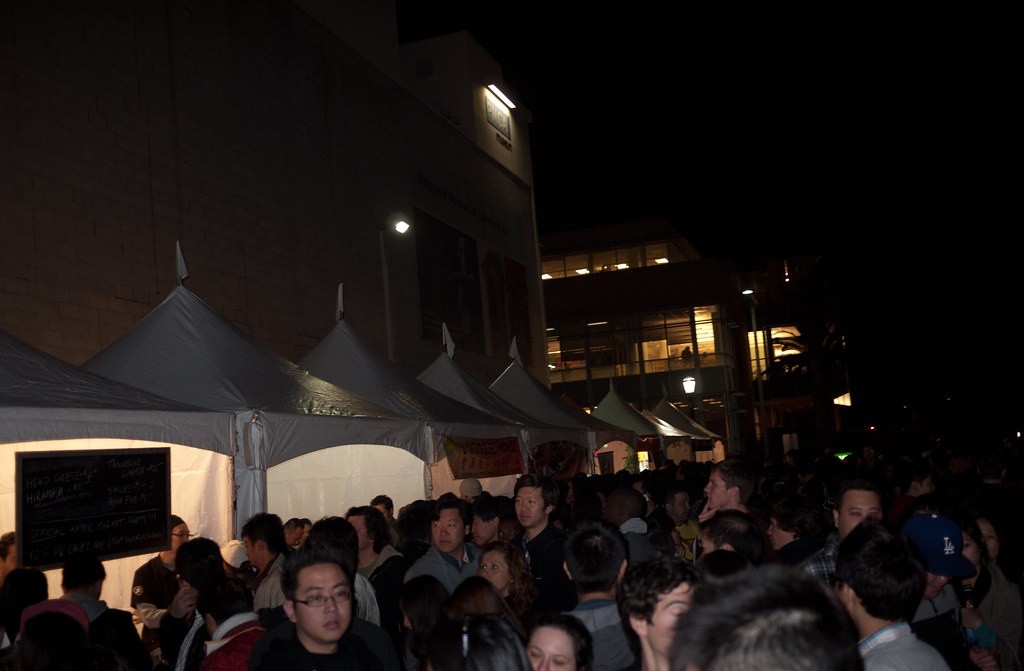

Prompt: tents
653;385;727;464
0;327;237;626
75;236;429;539
416;322;595;482
300;280;533;503
486;335;640;478
590;373;696;476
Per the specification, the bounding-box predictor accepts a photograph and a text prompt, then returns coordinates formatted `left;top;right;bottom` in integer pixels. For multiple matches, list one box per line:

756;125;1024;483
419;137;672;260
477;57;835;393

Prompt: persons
615;553;710;671
681;347;692;360
0;443;1024;671
669;563;868;671
520;614;595;671
247;541;403;671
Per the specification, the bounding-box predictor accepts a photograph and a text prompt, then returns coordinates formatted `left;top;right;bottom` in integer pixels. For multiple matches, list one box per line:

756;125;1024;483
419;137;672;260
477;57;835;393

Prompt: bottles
960;584;978;646
176;573;189;587
219;546;239;586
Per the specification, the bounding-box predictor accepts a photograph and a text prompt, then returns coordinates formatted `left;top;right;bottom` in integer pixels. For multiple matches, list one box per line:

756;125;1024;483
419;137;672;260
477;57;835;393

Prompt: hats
219;540;249;568
170;515;184;528
459;477;482;496
903;514;976;577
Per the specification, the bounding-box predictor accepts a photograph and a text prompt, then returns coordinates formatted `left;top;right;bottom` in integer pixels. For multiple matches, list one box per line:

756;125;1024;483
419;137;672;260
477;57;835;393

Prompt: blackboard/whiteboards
15;447;172;572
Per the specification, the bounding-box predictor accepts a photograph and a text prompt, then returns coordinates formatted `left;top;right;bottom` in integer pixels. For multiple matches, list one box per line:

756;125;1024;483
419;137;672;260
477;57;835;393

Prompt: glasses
286;592;352;607
172;533;194;540
